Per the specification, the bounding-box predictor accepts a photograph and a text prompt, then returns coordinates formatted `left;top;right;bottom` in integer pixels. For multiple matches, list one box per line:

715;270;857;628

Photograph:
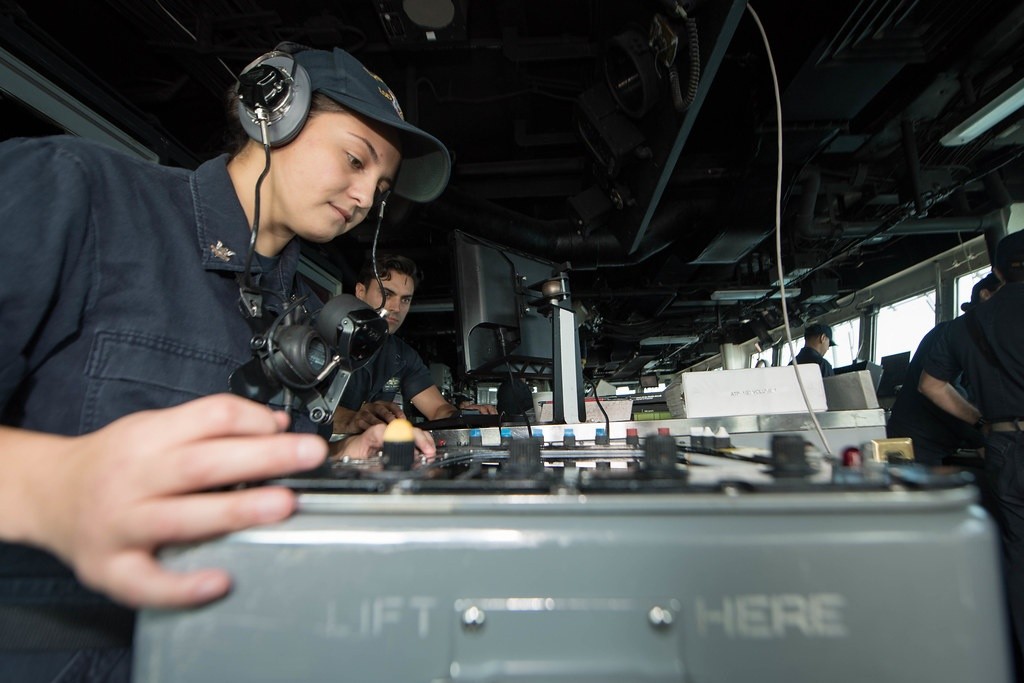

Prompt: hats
279;42;452;204
994;228;1024;270
804;321;836;346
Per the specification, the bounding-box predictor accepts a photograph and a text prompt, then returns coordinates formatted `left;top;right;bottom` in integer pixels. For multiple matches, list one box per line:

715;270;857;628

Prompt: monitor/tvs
451;229;572;380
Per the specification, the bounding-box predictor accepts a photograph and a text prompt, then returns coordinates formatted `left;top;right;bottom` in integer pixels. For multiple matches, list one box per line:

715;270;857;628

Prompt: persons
886;230;1024;683
788;323;838;377
0;41;498;683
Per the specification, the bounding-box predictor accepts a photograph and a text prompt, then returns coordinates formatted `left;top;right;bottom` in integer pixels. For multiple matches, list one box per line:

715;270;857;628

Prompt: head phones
231;41;315;149
